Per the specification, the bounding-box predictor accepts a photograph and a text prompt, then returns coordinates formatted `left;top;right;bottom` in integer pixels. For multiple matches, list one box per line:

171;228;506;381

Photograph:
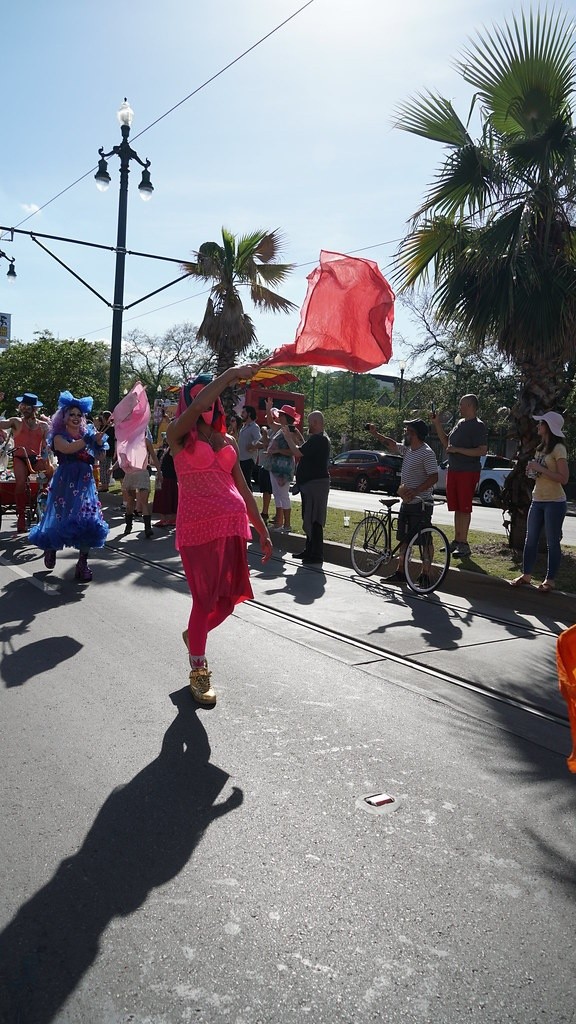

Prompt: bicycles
350;494;451;595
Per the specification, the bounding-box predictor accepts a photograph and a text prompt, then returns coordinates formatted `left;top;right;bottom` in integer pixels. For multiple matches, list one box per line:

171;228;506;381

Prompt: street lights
93;96;155;413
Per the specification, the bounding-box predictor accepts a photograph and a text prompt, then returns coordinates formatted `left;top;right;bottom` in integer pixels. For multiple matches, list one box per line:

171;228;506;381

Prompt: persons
0;392;54;531
429;394;488;558
28;388;110;582
363;419;439;589
90;406;163;537
154;396;332;564
166;364;274;705
504;410;570;592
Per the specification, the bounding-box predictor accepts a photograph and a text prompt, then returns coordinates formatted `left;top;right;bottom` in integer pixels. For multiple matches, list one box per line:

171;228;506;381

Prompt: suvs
327;449;404;493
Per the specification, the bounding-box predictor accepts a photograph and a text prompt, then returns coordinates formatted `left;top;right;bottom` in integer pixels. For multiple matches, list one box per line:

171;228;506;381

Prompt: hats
16;393;43;407
270;404;301;424
403;418;428;441
532;411;566;437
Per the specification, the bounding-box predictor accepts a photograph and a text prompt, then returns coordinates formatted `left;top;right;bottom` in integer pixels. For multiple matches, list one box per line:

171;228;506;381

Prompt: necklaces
197;428;215;448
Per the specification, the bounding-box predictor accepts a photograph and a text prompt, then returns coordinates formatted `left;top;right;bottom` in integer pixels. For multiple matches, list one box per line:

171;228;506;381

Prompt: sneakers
183;630;188;647
189;669;216;704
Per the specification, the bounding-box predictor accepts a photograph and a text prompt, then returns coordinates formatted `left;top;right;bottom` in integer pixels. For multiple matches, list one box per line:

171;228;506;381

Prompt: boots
143;515;153;536
124;514;134;533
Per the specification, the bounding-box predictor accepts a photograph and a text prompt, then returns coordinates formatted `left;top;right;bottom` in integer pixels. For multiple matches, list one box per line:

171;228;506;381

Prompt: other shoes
440;540;458;551
45;549;56;568
75;561;92;579
453;542;471;557
292;550;308;558
302;556;324;564
380;571;408;584
275;526;291;532
415;574;431;589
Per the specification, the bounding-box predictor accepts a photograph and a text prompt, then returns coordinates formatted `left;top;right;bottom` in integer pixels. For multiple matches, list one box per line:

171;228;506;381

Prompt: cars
434;454;517;507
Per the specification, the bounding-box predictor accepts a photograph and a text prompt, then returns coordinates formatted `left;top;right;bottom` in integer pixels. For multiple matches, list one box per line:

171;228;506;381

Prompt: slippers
510;577;529;585
537;584;556;592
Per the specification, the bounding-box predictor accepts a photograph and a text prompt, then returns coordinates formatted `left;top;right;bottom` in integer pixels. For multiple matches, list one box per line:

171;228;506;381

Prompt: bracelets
82;433;94;445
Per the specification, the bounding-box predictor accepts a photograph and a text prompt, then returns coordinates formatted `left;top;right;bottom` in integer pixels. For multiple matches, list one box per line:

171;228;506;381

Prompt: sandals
113;504;127;511
133;510;142;517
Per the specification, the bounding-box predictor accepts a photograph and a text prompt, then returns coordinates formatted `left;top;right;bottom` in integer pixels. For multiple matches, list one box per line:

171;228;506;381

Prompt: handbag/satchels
155;479;161;490
263;454;292;474
113;468;125;479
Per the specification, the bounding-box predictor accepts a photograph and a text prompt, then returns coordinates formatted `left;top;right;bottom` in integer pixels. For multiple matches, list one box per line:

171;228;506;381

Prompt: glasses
19;403;33;410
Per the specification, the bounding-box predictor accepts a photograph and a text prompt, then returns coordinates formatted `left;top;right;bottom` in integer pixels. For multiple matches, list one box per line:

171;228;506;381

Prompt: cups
527;460;537;478
343;516;350;528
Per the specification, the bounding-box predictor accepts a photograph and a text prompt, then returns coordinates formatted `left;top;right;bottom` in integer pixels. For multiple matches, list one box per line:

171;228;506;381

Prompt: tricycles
0;445;55;532
